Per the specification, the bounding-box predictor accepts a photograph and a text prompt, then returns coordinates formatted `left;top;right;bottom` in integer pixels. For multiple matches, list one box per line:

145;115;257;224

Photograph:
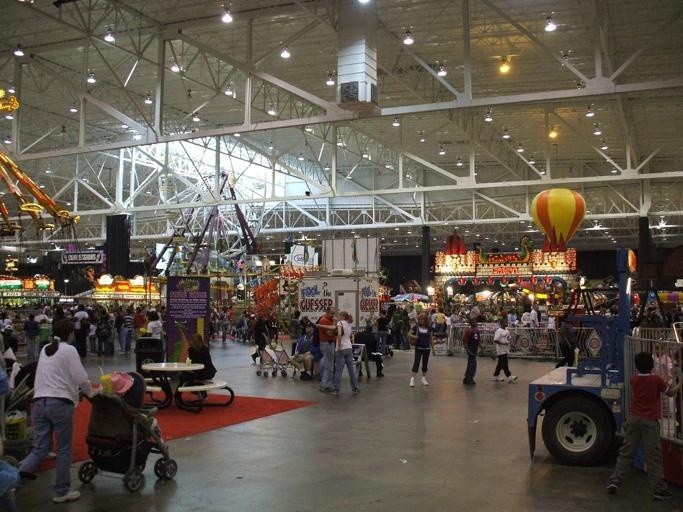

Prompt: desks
141;363;205;405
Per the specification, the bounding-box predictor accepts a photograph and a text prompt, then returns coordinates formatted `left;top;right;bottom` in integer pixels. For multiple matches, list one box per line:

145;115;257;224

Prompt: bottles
274;332;284;340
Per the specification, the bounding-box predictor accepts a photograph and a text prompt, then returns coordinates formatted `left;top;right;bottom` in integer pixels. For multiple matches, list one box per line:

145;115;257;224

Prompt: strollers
269;340;302;378
73;371;179;491
254;337;280;378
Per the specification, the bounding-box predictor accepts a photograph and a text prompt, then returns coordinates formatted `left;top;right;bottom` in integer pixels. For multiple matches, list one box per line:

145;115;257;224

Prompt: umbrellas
390;292;429;303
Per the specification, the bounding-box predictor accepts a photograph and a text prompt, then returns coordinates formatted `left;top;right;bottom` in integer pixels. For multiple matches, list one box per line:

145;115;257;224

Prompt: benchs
143;377;170;408
177;378;235;414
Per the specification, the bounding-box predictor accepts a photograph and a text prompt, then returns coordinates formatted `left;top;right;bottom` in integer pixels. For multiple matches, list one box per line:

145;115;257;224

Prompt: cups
185;359;190;367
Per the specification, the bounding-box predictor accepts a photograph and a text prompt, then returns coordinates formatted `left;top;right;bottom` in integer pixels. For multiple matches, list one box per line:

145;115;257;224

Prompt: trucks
526;249;683;491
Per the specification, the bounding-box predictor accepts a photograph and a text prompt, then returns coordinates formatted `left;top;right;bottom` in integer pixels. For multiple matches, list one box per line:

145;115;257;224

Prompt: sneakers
53;491;80;502
493;375;518;383
409;376;428;387
654;490;673;499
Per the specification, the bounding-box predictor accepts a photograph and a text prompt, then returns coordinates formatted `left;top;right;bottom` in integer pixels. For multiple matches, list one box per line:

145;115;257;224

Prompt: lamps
224;84;233;95
193;113;200;121
172;60;182;72
70;101;77;112
281;45;290;58
221;7;233;23
268;105;276;115
87;72;96;83
187;89;193;101
543;14;609;151
8;82;16;94
5;109;16;120
104;28;115;42
145;94;152;104
325;73;335;86
390;27;536;168
14;44;24;56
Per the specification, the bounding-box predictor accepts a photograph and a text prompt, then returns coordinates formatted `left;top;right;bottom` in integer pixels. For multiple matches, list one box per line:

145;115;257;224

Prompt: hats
4;325;12;331
111;373;134;395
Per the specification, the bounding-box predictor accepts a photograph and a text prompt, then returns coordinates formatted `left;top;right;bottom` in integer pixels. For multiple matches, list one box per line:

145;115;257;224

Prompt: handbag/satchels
333;321;344;336
409;328;418;346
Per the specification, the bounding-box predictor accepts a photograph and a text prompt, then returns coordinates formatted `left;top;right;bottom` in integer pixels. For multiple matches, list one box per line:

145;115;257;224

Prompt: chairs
308;342;374;382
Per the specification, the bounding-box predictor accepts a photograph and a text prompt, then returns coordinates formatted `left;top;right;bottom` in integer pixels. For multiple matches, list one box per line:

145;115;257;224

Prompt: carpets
30;381;319;473
